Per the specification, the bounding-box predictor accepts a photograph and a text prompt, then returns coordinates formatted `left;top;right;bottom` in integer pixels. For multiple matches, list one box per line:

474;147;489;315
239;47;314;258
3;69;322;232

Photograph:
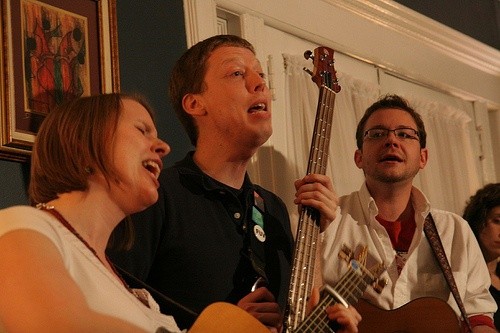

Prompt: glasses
358;127;423;148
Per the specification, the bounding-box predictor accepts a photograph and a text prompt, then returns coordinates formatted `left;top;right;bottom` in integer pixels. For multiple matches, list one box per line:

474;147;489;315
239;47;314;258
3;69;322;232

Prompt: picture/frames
0;0;121;164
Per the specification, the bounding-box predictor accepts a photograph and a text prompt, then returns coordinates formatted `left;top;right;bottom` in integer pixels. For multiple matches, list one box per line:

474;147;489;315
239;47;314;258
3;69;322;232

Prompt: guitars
330;295;462;333
185;245;388;333
276;47;341;333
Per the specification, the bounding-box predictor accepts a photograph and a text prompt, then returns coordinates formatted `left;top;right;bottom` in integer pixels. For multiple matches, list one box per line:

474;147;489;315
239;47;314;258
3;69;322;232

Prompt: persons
462;183;500;333
289;93;498;333
0;93;272;333
106;34;339;333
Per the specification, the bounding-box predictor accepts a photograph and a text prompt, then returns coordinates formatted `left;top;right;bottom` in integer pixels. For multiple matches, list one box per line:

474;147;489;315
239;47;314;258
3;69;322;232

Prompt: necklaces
37;203;133;296
